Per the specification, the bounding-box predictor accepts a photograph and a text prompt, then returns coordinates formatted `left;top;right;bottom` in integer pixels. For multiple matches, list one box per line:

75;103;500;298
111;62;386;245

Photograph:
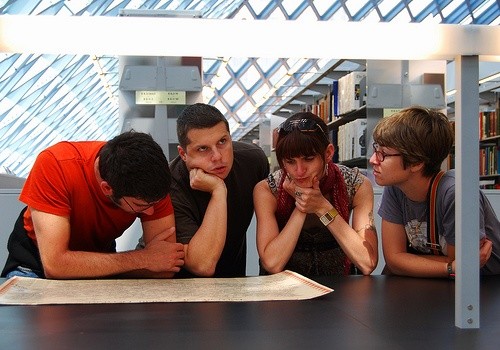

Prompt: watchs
320;208;338;226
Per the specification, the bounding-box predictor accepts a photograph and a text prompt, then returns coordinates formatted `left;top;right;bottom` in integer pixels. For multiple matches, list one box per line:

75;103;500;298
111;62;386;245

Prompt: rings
295;192;301;196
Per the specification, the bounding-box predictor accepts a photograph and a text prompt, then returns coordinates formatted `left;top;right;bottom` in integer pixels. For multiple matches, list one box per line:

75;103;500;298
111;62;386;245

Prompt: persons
0;132;184;280
370;107;500;277
171;103;271;279
252;112;379;276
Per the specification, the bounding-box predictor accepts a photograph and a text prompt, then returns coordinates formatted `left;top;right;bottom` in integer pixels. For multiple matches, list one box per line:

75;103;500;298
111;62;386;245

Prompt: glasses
372;143;404;162
123;194;167;213
279;119;326;137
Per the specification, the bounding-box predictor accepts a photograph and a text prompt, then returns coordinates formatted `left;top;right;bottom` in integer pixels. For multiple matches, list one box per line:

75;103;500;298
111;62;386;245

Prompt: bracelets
447;262;453;274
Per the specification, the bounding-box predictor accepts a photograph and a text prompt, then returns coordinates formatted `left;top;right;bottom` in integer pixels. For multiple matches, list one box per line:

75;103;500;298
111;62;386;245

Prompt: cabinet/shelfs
117;11;500;275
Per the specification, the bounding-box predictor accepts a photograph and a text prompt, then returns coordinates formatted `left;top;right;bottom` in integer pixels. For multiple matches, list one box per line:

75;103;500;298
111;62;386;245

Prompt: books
303;71;366;125
338;118;367;161
479;112;500;175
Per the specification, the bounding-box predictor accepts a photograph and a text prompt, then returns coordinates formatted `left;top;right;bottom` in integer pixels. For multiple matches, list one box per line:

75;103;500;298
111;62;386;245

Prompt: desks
0;275;500;350
